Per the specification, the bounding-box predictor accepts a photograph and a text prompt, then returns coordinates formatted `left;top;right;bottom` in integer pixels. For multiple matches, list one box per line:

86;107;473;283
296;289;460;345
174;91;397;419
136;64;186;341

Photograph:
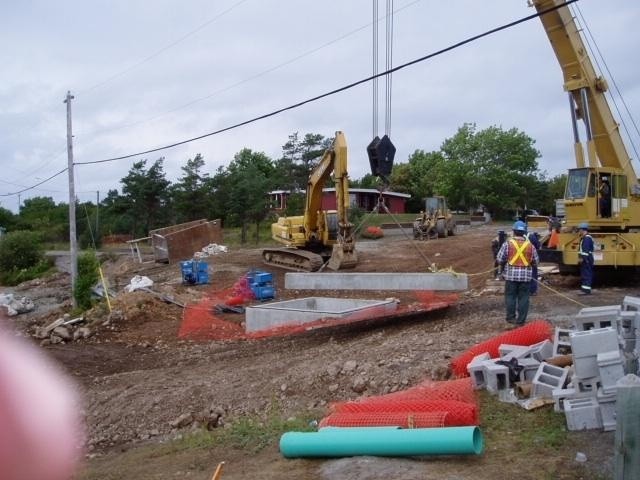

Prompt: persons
529;234;541;296
491;230;507;277
496;220;540;329
577;223;594;295
599;177;610;217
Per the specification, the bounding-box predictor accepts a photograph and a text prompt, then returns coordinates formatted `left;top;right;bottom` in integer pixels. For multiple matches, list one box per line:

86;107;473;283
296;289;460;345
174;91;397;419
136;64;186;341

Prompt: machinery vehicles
526;0;640;283
412;194;459;239
260;130;361;277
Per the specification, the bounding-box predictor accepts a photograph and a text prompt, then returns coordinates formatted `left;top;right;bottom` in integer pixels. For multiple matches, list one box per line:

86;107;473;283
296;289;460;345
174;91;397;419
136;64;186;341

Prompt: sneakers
505;321;524;330
576;290;592;295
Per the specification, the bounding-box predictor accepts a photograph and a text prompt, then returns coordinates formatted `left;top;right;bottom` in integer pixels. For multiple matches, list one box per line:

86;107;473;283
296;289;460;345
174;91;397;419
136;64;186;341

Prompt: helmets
577;223;589;230
511;220;528;232
